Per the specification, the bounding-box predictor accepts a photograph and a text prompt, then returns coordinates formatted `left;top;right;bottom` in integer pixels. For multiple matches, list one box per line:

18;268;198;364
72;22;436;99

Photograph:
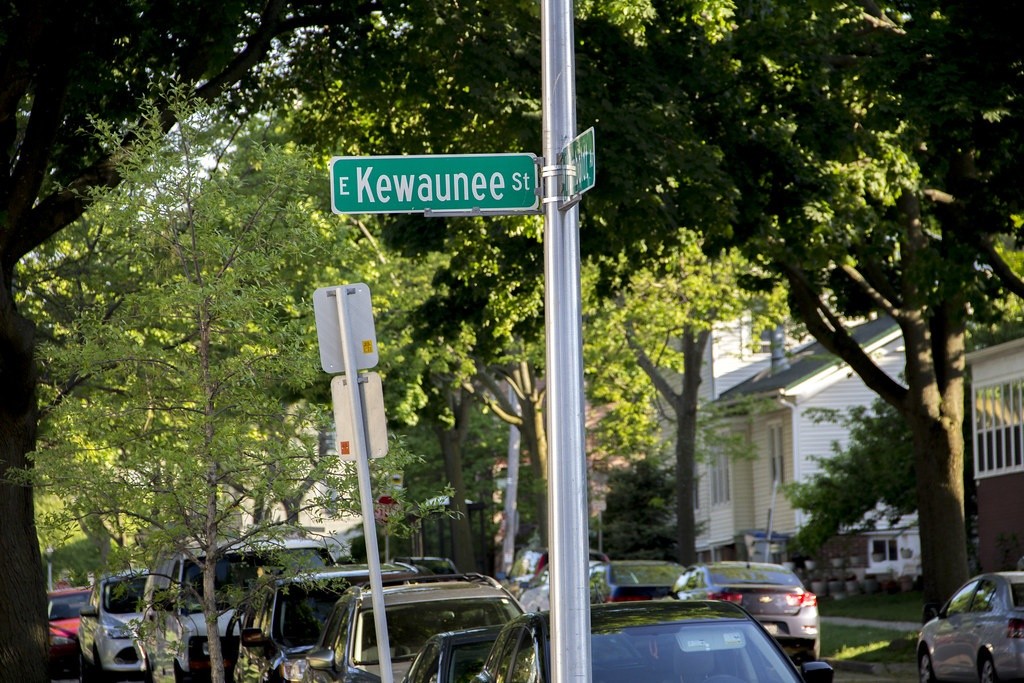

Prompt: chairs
674;643;715;683
51;603;72;620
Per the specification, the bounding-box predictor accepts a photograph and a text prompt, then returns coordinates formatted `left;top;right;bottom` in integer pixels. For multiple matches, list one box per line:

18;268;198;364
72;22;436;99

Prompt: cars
400;623;507;683
465;597;835;683
588;559;687;605
915;570;1024;683
45;585;92;678
74;524;613;683
302;579;528;683
664;561;823;662
